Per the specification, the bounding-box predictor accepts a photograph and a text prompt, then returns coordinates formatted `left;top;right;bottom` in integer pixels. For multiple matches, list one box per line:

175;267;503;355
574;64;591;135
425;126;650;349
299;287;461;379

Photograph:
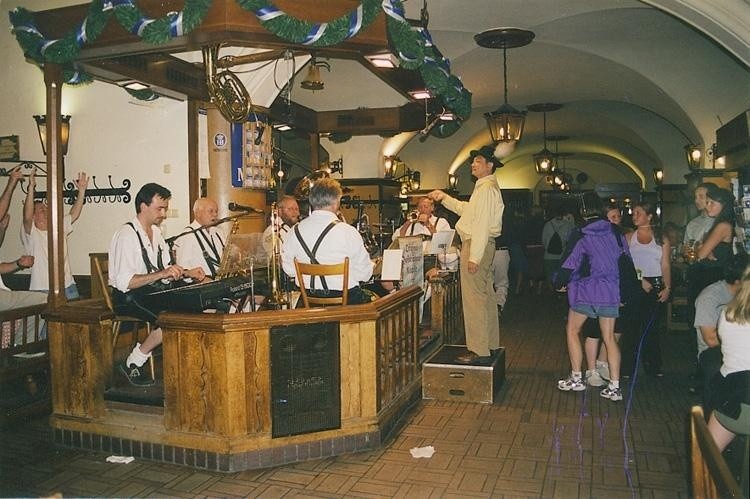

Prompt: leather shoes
454;350;490;366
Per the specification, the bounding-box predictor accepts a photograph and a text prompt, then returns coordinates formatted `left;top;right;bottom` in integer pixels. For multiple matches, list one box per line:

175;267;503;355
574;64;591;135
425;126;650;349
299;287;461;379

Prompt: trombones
268;202;287;304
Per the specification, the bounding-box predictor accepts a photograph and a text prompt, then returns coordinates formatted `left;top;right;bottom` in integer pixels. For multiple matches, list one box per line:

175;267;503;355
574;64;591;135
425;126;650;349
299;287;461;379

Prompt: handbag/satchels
618;254;640;302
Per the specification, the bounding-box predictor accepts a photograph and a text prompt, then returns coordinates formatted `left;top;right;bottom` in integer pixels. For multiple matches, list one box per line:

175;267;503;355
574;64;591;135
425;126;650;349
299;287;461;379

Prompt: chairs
294;258;350;308
652;248;749;499
92;256;158;384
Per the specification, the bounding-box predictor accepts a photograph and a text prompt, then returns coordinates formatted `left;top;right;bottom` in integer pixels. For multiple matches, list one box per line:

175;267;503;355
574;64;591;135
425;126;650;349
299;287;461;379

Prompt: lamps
685;143;704;171
298;54;332;92
526;101;562;174
399;170;420;194
546;133;575;198
383;155;405;179
32;112;74;196
450;173;460;191
652;167;665;182
473;25;537;145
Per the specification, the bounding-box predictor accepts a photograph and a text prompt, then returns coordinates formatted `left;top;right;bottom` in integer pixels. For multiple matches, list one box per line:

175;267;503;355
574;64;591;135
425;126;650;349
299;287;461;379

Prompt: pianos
146;266;275;314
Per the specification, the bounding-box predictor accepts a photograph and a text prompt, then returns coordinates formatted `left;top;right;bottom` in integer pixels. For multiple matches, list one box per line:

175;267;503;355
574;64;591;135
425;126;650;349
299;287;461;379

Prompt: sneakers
557;378;587;393
596;360;610;380
599;385;623;402
584;369;607;388
118;358;156;389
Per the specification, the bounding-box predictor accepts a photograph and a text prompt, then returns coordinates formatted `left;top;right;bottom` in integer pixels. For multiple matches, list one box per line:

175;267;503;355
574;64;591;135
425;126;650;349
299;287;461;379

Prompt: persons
491;207;574;321
280;178;376;307
429;147;506;361
390;196;450;320
553;193;679;402
106;182;206;387
683;182;750;451
175;197;266;313
0;167;89;359
254;196;300;265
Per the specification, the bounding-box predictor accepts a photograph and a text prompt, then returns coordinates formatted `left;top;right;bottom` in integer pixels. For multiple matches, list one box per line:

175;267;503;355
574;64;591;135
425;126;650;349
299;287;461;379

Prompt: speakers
270;319;342;439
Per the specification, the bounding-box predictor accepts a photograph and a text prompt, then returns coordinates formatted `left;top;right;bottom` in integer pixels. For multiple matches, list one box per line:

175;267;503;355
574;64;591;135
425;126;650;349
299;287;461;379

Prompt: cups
688;239;698;263
245;128;273;188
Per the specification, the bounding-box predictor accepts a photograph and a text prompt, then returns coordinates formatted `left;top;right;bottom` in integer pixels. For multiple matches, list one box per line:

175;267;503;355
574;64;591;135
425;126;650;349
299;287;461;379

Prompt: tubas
201;40;252;124
294;170;347;224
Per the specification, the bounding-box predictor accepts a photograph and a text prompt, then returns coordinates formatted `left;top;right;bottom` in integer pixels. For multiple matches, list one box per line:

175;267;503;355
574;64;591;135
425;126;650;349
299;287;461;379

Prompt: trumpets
407;209;420;222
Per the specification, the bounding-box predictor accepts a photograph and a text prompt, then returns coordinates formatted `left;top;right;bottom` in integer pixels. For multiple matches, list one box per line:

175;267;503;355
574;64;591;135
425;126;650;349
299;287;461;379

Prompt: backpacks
547;232;564;254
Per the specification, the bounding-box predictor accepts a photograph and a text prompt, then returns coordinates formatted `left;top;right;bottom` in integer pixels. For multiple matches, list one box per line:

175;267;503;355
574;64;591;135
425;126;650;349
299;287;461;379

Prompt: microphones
228;202;264;213
255;126;266;145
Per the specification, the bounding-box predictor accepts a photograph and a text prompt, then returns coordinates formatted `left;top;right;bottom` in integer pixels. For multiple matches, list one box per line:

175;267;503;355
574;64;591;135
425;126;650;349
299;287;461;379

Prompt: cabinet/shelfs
336;178;410;254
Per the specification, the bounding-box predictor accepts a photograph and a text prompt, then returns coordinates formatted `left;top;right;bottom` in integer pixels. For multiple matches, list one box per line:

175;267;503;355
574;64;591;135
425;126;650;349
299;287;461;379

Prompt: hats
470;145;505;170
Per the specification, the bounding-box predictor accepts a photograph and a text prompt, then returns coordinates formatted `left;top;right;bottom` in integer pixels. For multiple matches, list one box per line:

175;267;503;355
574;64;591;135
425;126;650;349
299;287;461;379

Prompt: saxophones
215;220;247;277
